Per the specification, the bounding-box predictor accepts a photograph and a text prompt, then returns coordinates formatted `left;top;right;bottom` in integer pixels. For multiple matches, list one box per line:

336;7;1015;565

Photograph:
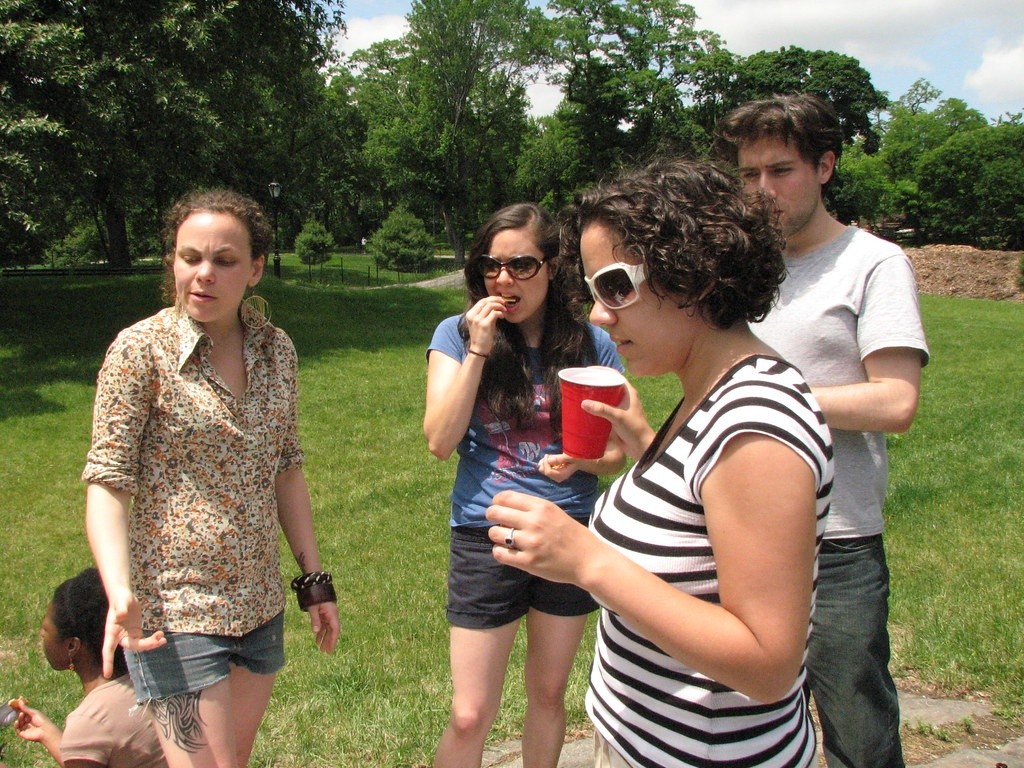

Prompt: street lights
268;179;283;277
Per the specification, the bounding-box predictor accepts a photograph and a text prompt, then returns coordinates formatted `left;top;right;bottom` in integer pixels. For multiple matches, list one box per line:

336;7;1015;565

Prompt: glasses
478;254;548;280
584;261;656;309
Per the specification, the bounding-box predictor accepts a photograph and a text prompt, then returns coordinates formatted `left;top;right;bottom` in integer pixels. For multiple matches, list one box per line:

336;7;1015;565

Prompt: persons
422;203;626;768
724;94;930;768
362;237;366;250
0;568;169;768
486;160;836;768
82;192;338;768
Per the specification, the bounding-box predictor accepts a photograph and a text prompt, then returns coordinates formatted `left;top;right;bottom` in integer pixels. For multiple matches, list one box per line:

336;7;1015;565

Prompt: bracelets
468;350;489;359
291;571;337;611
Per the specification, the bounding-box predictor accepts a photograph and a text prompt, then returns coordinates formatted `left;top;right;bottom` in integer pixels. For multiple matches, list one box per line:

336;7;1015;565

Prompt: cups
557;368;627;459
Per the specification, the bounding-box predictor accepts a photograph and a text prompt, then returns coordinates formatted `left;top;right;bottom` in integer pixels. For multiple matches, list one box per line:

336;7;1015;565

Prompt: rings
505;528;515;549
